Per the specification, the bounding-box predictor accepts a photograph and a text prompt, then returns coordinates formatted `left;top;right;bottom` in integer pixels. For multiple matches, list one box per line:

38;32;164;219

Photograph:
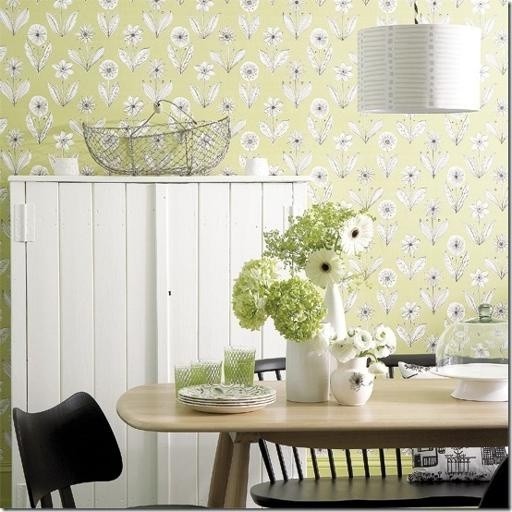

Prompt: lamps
356;2;484;116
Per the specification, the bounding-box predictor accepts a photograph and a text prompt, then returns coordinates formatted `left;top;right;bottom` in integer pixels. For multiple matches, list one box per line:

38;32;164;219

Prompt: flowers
331;325;395;363
263;199;374;288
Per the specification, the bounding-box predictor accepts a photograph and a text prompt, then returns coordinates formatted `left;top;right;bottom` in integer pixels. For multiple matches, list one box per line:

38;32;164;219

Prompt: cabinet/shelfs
9;173;311;507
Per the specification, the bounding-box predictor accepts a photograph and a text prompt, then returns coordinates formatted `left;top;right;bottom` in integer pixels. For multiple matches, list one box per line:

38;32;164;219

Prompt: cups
54;157;79;177
225;345;255;385
175;363;206;398
192;361;223;384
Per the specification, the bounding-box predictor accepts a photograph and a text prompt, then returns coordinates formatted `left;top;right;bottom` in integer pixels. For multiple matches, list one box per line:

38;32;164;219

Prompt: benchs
250;354;506;507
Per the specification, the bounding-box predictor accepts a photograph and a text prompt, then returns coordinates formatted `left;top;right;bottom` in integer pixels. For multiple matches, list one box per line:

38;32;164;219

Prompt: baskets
82;98;233;176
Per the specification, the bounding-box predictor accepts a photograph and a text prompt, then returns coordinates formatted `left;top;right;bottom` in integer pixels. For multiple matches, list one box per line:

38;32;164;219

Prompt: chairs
10;393;122;509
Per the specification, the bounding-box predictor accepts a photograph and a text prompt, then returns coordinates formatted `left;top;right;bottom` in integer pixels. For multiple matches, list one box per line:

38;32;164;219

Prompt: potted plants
229;257;329;403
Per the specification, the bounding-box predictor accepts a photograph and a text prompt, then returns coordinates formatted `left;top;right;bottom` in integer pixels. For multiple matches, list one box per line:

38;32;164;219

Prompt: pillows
397;362;508;484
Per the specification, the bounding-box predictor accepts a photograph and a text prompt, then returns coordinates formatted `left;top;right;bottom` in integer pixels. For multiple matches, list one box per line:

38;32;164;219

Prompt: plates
176;383;277;414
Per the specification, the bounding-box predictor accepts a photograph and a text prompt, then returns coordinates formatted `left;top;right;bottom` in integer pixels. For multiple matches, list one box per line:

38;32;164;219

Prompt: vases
329;358;373;404
322;286;348;339
